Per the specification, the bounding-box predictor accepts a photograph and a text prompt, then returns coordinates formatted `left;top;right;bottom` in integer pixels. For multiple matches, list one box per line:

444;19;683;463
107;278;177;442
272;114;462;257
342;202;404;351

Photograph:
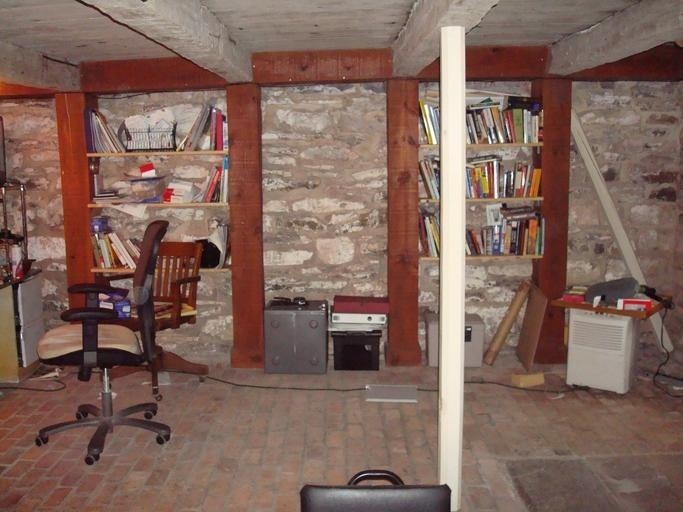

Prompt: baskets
123;120;177;152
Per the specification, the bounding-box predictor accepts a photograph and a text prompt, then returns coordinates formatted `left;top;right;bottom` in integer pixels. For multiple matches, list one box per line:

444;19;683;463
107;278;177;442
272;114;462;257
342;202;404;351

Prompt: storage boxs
330;331;383;372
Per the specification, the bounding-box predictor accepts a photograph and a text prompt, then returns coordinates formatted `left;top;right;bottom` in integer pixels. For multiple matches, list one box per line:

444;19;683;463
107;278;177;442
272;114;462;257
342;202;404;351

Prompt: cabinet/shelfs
84;145;232;280
415;138;546;264
0;172;28;279
1;268;45;387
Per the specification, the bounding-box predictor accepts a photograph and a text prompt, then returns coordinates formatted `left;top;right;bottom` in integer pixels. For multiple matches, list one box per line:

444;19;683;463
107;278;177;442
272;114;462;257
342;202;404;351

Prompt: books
82;104;230;326
332;295;391;315
416;87;545;258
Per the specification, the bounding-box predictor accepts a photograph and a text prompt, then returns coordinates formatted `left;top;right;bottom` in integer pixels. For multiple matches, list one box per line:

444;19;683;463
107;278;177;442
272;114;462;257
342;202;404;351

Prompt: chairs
298;467;453;511
98;238;210;380
33;218;176;468
